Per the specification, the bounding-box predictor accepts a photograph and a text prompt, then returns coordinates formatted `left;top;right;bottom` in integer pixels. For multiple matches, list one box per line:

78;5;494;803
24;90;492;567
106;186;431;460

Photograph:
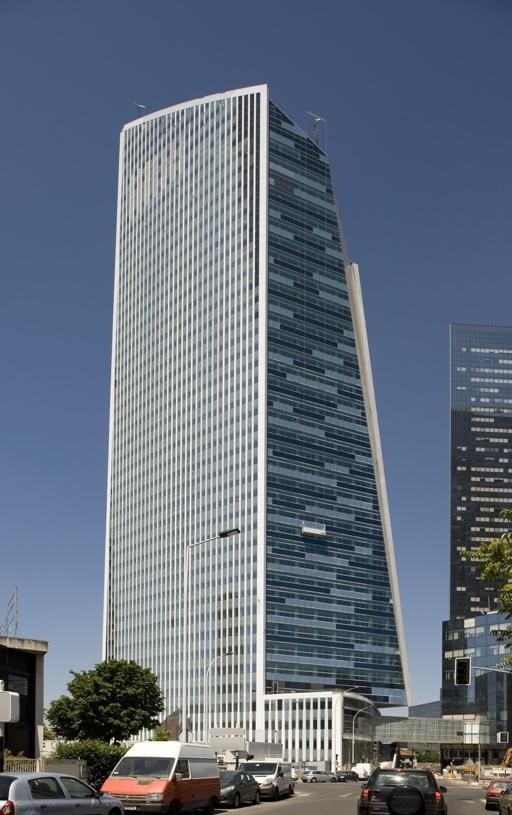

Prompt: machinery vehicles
499;746;512;769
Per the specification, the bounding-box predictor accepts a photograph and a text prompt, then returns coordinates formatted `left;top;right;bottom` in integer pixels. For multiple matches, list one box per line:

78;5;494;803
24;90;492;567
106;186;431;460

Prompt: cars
292;772;299;781
217;768;263;810
498;780;512;815
301;770;336;783
335;770;360;782
481;777;512;809
0;769;126;815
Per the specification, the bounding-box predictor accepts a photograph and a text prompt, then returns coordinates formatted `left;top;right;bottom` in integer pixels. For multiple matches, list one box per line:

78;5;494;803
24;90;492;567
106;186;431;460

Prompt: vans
236;760;297;801
97;738;223;815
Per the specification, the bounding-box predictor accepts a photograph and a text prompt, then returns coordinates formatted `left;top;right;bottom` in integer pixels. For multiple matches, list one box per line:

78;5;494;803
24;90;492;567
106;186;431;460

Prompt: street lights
350;704;374;764
234;749;240;771
182;527;241;742
333;684;360;773
205;652;234;743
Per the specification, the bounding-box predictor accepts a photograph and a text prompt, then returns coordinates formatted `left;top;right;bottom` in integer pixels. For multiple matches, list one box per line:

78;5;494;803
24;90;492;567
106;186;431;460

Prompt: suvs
354;764;449;815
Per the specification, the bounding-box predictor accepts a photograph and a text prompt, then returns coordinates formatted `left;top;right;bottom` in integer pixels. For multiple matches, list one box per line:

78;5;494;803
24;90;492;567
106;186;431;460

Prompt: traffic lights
454;656;473;687
